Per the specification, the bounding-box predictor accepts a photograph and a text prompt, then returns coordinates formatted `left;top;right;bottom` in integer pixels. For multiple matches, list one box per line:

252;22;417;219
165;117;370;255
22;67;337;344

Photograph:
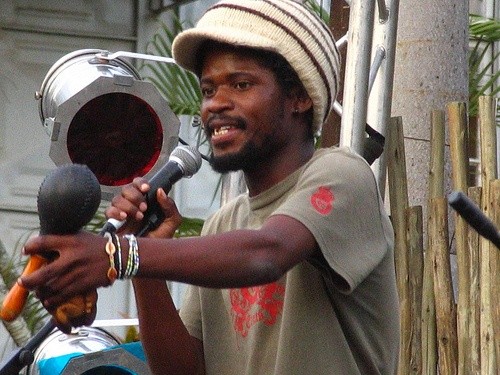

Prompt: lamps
34;46;180;195
25;328;152;375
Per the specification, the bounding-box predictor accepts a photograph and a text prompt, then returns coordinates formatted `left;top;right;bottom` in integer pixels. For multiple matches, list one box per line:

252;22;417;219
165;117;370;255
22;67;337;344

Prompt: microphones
100;146;202;240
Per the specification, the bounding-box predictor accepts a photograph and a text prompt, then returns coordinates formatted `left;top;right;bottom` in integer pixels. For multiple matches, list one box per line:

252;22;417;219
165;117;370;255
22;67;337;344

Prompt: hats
170;0;342;141
